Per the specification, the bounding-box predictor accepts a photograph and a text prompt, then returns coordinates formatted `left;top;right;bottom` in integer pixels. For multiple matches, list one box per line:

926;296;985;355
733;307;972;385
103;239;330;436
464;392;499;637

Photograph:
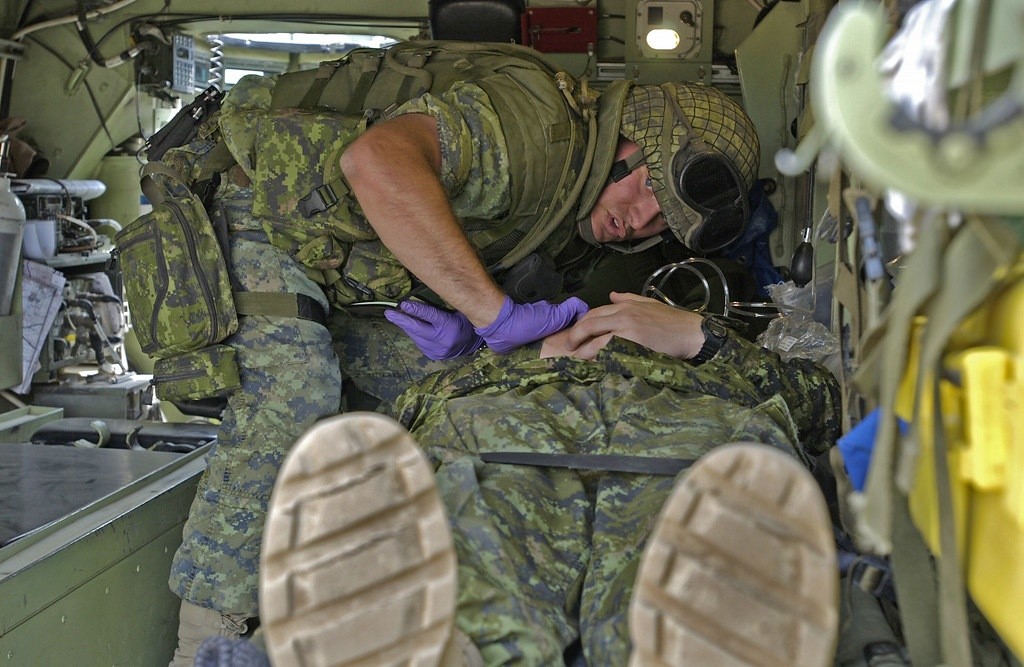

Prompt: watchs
681;308;728;367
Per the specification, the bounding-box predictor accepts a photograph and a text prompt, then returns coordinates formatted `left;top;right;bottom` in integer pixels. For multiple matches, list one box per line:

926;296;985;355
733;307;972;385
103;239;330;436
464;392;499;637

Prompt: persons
139;37;844;667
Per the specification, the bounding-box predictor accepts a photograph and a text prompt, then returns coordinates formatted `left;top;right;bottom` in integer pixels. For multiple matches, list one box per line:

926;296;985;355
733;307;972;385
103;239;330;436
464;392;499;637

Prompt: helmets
619;81;761;246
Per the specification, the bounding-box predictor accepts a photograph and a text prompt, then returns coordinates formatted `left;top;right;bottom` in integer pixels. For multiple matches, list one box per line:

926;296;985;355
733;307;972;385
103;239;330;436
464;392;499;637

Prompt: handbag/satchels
115;194;239;358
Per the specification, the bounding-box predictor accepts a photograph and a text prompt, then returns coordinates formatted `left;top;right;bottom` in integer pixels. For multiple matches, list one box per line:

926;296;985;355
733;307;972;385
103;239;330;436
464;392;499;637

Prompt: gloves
384;300;484;360
475;295;590;354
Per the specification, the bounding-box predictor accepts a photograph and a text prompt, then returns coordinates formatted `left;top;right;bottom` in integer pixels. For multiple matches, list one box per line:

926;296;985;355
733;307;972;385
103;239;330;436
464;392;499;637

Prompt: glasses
660;81;751;256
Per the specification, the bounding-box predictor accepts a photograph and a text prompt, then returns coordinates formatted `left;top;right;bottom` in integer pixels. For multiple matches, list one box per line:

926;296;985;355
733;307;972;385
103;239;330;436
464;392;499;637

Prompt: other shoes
630;441;839;667
170;599;248;667
259;411;458;667
